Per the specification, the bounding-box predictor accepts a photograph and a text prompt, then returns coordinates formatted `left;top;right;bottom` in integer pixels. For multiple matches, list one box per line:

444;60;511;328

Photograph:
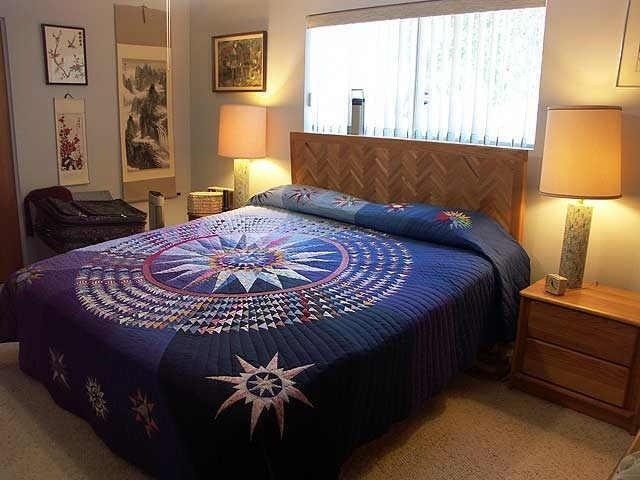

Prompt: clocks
544;274;566;296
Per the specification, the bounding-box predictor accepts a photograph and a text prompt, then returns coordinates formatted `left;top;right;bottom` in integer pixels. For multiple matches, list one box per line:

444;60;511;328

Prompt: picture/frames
616;1;640;88
210;30;267;93
41;23;88;86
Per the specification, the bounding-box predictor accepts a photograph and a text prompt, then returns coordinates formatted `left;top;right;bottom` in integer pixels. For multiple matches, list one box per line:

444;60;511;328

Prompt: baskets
186;191;222;214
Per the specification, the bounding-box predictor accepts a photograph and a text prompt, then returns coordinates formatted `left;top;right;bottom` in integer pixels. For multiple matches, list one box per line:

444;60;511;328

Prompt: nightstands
186;208;228;221
507;274;639;435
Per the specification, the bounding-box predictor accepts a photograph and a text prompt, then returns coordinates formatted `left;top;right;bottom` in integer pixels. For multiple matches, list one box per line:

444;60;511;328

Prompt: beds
4;131;529;479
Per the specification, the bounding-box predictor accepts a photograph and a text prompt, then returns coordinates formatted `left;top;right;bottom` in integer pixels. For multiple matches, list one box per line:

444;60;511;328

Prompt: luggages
33;197;147;251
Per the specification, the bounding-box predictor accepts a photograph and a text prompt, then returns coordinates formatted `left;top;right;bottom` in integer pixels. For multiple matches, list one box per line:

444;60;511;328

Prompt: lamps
537;104;623;288
218;105;267;210
